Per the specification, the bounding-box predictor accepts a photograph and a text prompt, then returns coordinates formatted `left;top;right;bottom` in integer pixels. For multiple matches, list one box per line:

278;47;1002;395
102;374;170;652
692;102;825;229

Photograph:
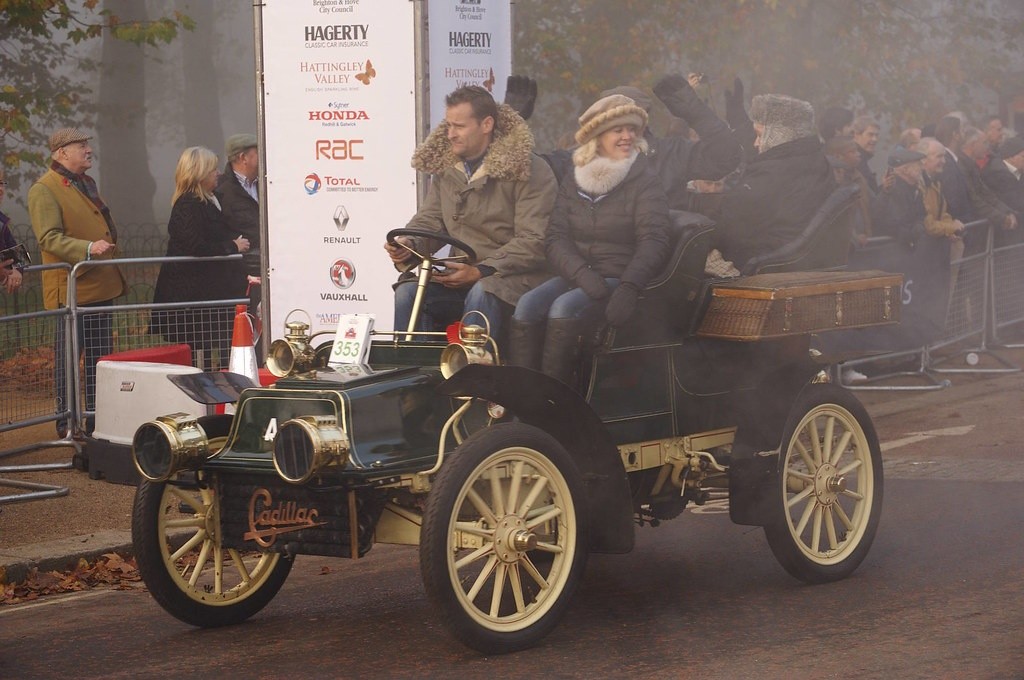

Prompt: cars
130;176;903;659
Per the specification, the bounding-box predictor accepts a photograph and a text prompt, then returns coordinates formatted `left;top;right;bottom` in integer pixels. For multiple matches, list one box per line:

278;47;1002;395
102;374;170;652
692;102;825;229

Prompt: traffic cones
220;303;262;421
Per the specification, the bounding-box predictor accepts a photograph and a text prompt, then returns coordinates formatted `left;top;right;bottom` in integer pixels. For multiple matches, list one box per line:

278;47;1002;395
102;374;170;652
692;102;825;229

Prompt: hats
48;128;93;152
600;86;651;112
748;94;826;153
574;94;649;168
227;134;258;157
887;149;926;167
1001;138;1024;159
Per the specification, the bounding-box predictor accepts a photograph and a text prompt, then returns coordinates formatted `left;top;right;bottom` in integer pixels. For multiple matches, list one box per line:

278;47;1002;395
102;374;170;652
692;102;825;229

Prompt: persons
146;133;261;370
507;94;673;390
27;127;127;439
383;85;561;353
0;162;24;295
504;71;1024;385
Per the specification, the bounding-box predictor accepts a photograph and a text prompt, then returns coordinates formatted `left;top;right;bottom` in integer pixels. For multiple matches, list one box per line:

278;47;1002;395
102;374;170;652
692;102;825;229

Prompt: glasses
0;180;7;186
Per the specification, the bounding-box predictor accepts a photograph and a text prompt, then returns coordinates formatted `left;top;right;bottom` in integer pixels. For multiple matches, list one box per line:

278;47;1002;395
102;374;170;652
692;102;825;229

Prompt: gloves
652;72;728;140
576;269;610;299
503;75;537;119
725;78;777;150
605;284;639;328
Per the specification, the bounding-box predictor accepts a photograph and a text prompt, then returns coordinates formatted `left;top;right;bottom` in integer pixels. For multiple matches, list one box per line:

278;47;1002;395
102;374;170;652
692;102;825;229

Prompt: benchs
575;208;718;347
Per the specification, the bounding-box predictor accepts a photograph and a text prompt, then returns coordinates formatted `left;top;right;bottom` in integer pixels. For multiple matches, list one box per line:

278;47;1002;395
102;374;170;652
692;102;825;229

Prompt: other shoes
60;426;89;442
